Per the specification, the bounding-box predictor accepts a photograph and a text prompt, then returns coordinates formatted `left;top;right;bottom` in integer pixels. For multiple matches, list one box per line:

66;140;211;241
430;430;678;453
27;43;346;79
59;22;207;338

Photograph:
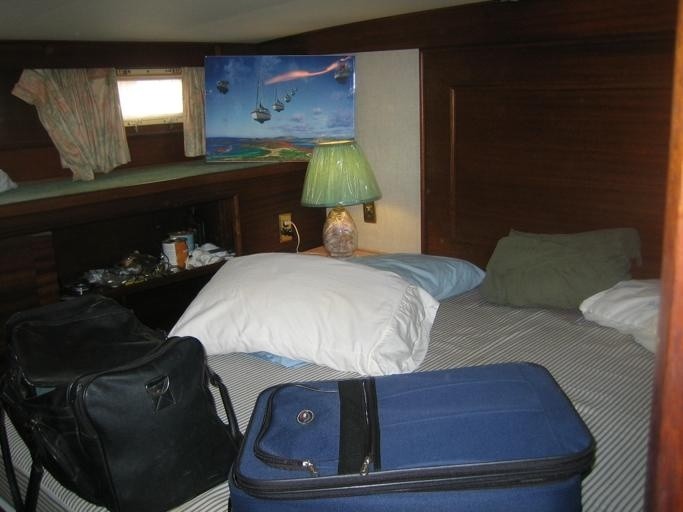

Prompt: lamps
301;139;382;257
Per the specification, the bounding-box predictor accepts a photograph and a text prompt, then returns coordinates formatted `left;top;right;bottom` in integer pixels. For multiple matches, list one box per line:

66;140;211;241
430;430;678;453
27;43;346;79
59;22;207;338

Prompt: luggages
228;359;597;512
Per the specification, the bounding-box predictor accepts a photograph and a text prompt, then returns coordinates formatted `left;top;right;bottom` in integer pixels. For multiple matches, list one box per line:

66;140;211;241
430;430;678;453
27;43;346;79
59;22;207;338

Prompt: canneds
161;230;197;269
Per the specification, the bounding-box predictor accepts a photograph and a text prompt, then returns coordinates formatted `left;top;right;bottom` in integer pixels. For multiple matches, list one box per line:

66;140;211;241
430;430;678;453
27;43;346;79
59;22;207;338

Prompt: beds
0;240;662;512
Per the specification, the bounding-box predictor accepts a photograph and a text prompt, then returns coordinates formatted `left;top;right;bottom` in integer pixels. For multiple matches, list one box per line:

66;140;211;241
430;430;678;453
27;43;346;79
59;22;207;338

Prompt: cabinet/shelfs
0;230;61;319
190;172;324;253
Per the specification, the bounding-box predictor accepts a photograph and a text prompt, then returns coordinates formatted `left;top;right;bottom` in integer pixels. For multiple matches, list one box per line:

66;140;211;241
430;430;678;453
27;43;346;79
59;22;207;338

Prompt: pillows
252;254;486;368
480;232;642;312
166;254;439;375
580;279;658;356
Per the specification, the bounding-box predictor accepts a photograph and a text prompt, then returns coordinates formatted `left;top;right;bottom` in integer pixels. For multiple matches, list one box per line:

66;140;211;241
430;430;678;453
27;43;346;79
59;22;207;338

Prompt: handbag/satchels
0;291;250;512
